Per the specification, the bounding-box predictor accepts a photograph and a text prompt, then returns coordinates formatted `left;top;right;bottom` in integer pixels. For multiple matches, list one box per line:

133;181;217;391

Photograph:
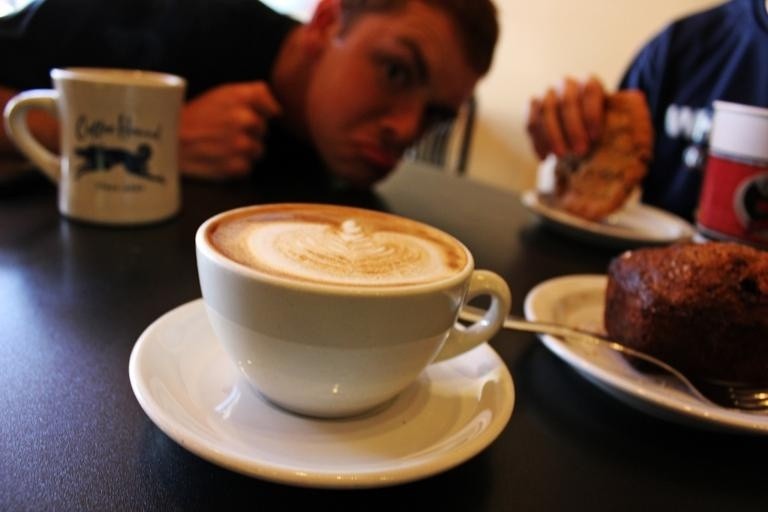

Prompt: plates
520;273;767;438
520;188;695;249
126;296;517;489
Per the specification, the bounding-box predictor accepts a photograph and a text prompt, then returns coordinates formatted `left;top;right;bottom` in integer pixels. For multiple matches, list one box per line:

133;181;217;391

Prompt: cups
694;96;768;252
196;201;513;422
2;68;189;226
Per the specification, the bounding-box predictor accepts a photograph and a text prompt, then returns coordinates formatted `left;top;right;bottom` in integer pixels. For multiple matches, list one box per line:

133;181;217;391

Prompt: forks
454;302;768;410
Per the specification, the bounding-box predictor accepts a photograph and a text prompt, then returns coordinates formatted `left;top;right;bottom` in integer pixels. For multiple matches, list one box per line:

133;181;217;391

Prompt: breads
554;85;656;222
603;240;768;388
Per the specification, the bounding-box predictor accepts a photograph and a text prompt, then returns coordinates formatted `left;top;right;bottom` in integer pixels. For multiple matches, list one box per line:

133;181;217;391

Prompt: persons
0;0;501;192
526;0;768;236
